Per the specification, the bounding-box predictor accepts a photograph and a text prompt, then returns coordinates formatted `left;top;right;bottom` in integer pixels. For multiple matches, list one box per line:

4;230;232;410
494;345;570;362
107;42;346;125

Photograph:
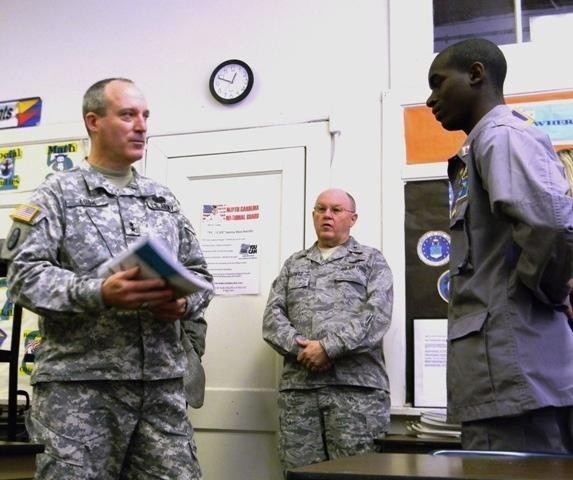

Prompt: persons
262;189;396;479
425;40;573;456
6;78;214;479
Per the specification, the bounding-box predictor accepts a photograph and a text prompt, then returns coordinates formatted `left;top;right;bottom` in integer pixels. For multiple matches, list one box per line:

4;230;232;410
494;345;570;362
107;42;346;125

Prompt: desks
372;433;462;453
0;441;45;480
283;451;573;480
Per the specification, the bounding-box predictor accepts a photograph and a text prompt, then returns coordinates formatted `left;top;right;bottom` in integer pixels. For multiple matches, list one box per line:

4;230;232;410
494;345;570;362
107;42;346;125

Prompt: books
406;412;462;441
98;238;213;310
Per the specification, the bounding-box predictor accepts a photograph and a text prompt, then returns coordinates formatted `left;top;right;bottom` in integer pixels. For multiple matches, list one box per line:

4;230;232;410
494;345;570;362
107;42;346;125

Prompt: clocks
210;59;253;105
